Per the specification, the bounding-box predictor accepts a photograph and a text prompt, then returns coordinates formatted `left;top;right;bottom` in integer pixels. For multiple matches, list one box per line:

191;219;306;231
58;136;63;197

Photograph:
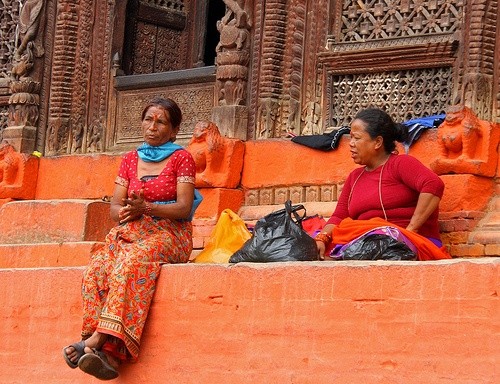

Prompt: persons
312;108;445;260
63;98;197;380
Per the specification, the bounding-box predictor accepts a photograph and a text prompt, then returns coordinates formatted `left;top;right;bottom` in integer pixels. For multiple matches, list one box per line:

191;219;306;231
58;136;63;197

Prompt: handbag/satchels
229;200;318;262
189;208;252;264
342;233;416;261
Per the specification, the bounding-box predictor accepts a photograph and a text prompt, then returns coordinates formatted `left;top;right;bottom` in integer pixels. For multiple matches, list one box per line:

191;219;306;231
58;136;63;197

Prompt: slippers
78;347;119;380
63;340;85;369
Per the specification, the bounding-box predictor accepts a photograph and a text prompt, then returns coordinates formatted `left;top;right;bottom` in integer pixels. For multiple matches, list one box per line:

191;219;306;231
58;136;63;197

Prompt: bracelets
314;233;331;244
143;203;152;216
